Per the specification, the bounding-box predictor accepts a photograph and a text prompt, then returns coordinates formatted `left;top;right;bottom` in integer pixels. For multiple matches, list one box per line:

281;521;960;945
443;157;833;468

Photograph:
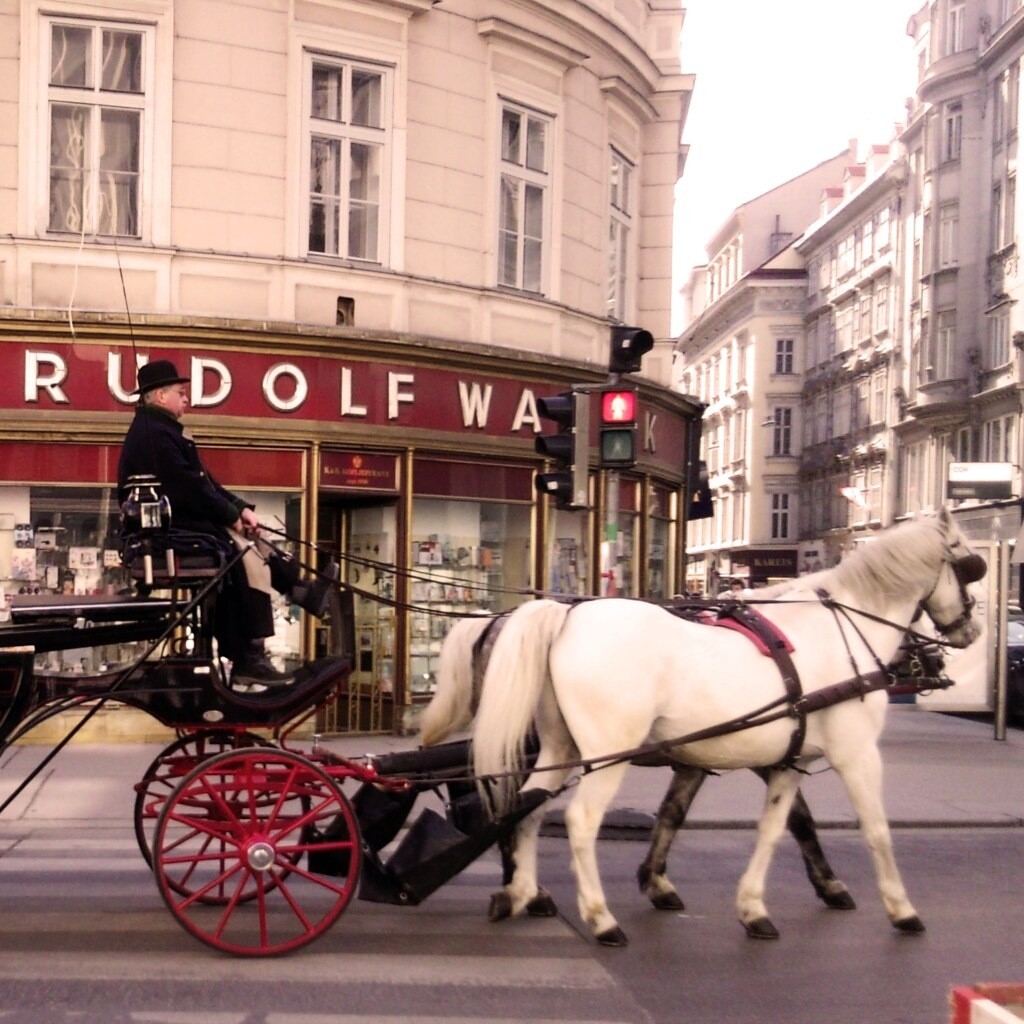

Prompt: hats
128;359;191;395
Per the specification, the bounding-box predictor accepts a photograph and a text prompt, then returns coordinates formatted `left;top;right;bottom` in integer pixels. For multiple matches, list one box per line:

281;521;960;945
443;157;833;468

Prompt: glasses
169;386;186;395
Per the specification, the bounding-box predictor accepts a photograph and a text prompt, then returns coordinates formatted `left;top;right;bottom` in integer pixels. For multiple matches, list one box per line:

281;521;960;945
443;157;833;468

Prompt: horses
413;504;990;947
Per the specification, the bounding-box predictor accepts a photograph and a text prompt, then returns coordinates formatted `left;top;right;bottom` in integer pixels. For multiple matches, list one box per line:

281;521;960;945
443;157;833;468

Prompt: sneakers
230;645;298;687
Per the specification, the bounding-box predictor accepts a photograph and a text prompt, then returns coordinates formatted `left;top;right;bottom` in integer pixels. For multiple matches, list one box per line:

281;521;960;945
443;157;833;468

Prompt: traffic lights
601;392;638;472
533;394;577;509
610;324;655;375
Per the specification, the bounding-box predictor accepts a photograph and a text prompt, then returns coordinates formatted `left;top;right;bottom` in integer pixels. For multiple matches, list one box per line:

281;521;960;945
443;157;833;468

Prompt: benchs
114;472;231;601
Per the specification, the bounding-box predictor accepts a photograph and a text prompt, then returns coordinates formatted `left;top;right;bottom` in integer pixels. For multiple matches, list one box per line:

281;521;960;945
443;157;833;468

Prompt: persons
730;580;744;590
117;359;340;690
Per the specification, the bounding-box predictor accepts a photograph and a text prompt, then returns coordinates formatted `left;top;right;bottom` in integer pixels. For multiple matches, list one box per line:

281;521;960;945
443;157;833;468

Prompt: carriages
0;507;984;955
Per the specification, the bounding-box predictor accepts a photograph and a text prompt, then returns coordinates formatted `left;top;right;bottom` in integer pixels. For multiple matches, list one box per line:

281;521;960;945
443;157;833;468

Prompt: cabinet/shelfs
381;556;504;657
